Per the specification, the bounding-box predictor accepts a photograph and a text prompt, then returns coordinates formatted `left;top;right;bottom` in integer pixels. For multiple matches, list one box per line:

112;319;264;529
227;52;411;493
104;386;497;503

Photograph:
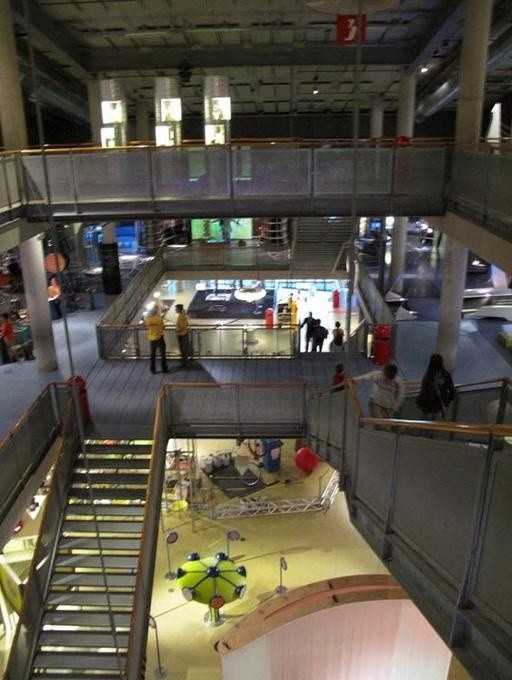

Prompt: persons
174;304;191;372
363;227;383;240
299;311;344;353
48;276;62;319
330;364;349;392
416;356;456;439
164;446;218;509
145;305;170;374
352;364;404;431
278;290;293;312
0;251;23;366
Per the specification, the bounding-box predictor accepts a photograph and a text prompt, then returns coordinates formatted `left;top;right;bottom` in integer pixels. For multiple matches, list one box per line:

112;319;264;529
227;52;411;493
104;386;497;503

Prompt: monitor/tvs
369;220;383;231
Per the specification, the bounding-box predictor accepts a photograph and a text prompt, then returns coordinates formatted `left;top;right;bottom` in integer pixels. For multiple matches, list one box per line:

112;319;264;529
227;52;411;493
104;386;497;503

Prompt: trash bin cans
265;308;274;329
262;439;283;473
333;290;340;307
67;376;92;423
373;324;391;365
